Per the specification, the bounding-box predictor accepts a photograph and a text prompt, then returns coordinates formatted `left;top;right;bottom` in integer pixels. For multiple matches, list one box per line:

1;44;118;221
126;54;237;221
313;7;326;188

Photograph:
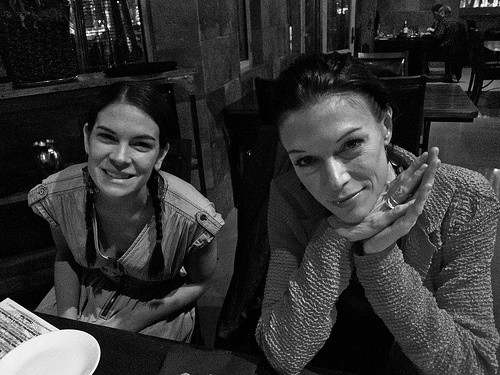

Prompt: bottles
403;20;408;34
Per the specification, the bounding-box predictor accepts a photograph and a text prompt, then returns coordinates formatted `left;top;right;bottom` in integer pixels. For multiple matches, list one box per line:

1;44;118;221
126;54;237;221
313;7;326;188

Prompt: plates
0;330;100;375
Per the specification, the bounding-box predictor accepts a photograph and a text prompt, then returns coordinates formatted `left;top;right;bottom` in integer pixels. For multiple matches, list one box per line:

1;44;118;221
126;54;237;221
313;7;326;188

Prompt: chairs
357;52;409;78
373;74;433;163
466;26;500;109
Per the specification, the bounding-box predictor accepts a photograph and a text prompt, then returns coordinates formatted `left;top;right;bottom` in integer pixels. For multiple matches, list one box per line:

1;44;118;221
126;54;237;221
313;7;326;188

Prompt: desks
0;297;357;375
219;80;482;163
